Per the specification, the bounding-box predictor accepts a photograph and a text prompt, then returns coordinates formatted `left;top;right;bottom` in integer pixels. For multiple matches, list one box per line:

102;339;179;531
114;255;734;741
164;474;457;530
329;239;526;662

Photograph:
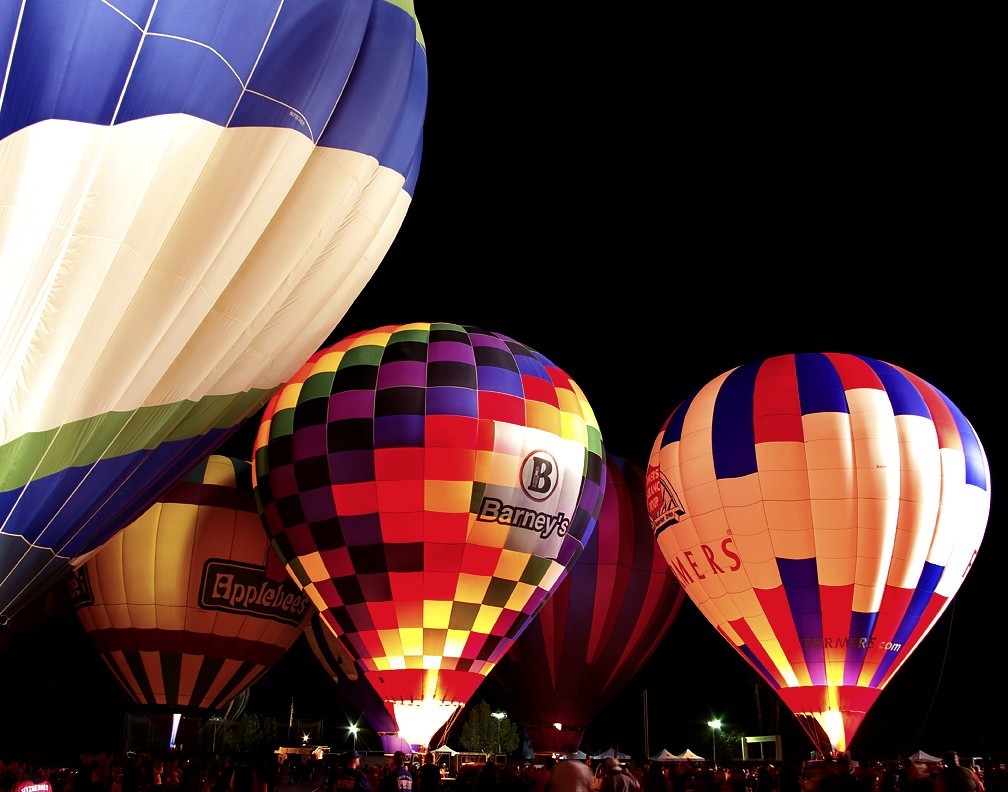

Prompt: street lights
490;711;504;754
350;725;358;752
706;720;722;766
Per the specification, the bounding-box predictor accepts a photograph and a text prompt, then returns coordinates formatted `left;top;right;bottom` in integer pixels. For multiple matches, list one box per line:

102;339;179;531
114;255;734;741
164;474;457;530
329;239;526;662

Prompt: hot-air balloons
303;613;373;711
0;2;432;633
479;449;685;766
644;352;993;792
72;450;317;765
251;319;608;772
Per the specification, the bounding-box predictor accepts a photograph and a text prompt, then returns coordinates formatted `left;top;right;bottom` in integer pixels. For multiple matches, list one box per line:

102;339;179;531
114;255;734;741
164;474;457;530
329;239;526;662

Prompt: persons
0;744;1008;792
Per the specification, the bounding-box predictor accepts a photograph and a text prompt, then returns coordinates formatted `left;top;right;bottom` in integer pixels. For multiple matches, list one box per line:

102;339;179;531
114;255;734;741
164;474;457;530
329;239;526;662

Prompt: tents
593;748;630;759
552;749;592;759
678;749;705;764
651;748;681;763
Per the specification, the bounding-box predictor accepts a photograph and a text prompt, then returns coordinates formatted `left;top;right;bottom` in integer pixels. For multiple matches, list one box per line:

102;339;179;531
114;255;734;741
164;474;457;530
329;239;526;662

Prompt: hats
606;757;624;771
